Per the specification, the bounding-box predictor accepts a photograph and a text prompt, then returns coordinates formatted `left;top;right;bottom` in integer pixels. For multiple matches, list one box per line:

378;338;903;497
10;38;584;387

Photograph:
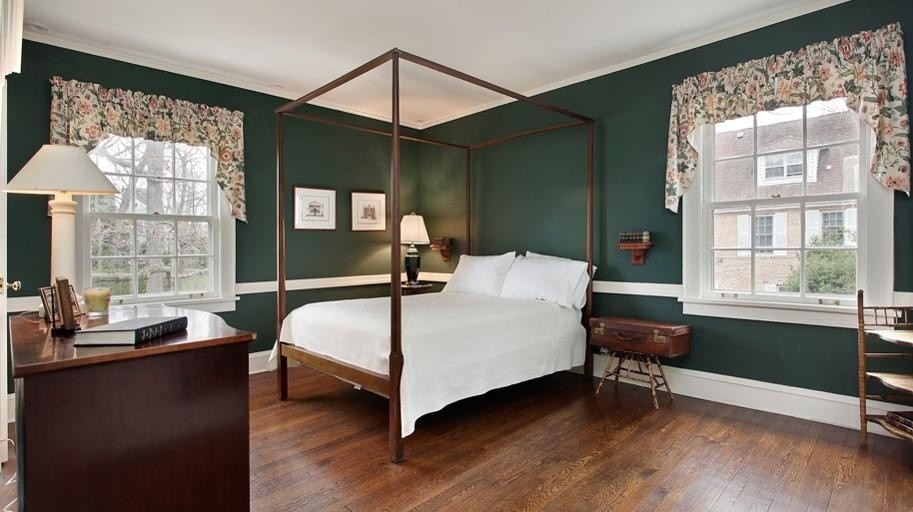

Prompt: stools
595;348;675;410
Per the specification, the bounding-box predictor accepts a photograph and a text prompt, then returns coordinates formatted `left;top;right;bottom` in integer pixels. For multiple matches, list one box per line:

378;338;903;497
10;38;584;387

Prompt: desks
9;304;258;512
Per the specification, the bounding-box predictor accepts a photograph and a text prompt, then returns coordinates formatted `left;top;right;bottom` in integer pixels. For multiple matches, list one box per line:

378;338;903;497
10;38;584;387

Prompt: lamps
399;211;430;285
0;143;120;301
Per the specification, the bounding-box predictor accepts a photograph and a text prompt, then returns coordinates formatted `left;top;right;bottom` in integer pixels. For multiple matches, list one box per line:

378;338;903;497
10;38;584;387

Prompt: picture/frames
350;192;387;231
38;284;83;323
292;186;337;231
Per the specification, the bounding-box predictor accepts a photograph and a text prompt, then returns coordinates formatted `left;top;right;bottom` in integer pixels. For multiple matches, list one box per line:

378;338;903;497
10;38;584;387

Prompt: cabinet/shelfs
857;288;913;449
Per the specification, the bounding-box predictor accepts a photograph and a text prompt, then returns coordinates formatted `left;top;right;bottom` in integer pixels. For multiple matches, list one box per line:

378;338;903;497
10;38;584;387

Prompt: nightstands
398;283;433;296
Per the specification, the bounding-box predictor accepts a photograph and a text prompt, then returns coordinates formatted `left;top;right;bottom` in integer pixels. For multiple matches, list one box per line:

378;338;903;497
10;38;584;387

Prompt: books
72;315;187;347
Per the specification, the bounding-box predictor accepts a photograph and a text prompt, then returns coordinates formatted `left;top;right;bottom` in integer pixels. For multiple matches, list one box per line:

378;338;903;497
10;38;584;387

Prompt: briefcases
588;315;691;358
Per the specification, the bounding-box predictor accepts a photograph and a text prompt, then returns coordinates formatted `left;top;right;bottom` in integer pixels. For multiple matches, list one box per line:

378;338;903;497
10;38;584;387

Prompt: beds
274;47;596;463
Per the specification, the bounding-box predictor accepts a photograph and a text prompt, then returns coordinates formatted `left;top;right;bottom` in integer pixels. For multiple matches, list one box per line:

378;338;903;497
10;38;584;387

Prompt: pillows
440;251;598;307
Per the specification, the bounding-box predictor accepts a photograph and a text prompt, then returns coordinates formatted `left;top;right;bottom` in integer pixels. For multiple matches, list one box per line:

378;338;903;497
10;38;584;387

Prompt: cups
79;286;113;318
